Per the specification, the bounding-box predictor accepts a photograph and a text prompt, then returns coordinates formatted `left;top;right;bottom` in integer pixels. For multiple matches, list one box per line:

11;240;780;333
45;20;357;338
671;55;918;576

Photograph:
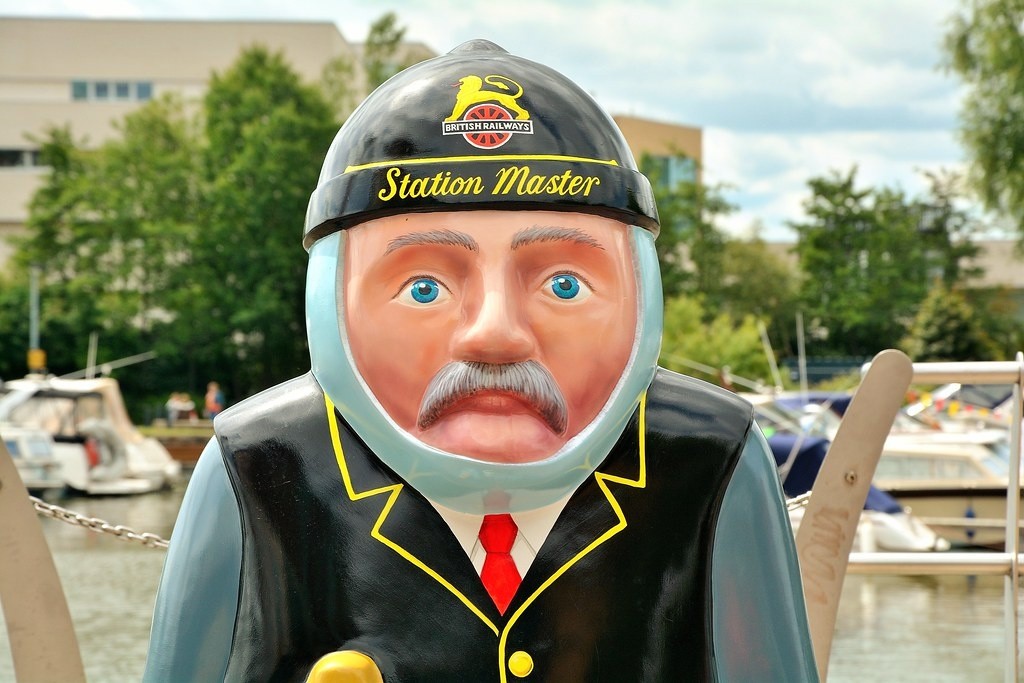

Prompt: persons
206;383;222;421
140;39;818;683
164;392;200;428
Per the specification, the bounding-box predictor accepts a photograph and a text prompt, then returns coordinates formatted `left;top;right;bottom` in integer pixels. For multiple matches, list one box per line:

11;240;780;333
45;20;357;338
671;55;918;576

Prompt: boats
739;384;1023;553
0;267;182;504
